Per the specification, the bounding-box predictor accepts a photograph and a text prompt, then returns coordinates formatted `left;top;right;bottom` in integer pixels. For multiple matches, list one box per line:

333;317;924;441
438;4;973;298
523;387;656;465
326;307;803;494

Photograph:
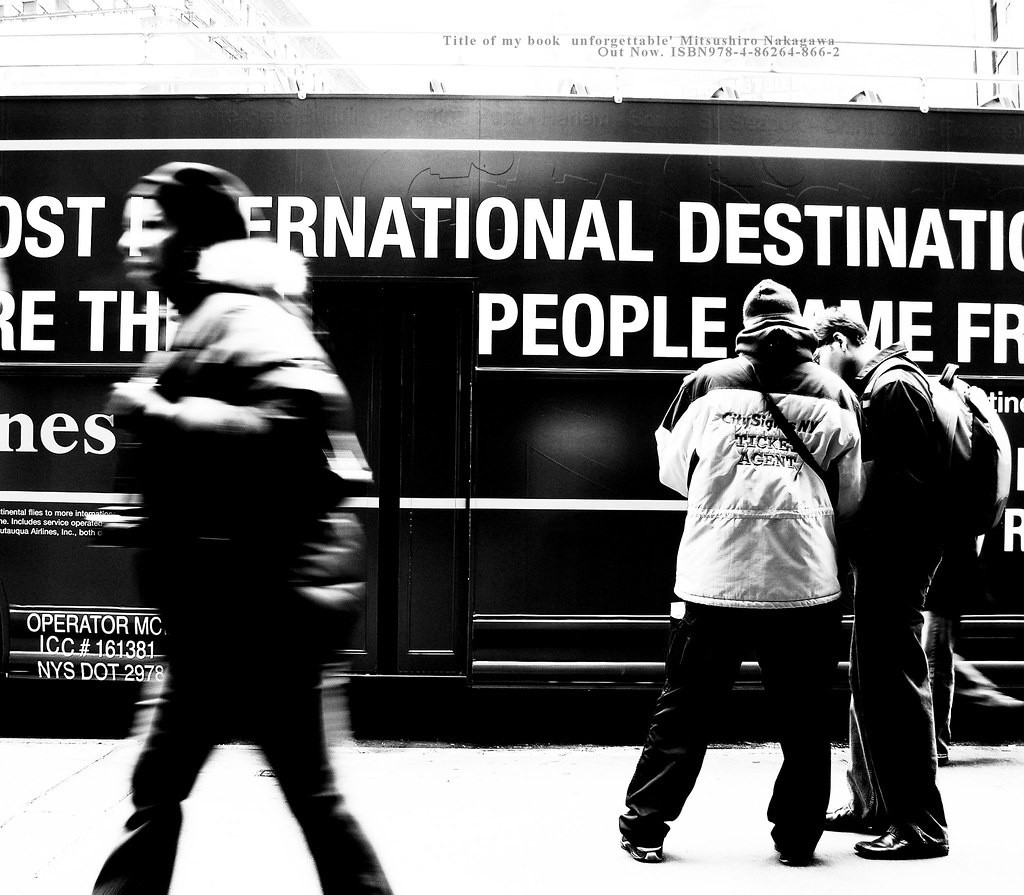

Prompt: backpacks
862;361;1012;535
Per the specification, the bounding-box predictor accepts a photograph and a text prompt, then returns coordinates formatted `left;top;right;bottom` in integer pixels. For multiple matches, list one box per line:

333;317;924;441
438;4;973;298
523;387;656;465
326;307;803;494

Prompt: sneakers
620;834;662;861
779;853;814;864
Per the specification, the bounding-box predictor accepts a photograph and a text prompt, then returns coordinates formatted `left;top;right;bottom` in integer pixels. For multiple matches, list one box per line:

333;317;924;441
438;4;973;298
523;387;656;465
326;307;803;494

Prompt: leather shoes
824;804;889;835
854;832;948;859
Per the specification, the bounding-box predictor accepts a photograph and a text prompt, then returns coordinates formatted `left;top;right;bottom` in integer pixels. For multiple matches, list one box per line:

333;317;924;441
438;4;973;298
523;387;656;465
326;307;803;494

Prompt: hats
125;160;258;237
743;279;802;328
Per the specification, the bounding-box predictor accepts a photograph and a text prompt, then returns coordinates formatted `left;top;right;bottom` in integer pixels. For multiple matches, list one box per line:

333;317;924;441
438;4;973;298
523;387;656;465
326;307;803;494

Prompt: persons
95;160;394;895
618;278;868;867
811;305;953;860
